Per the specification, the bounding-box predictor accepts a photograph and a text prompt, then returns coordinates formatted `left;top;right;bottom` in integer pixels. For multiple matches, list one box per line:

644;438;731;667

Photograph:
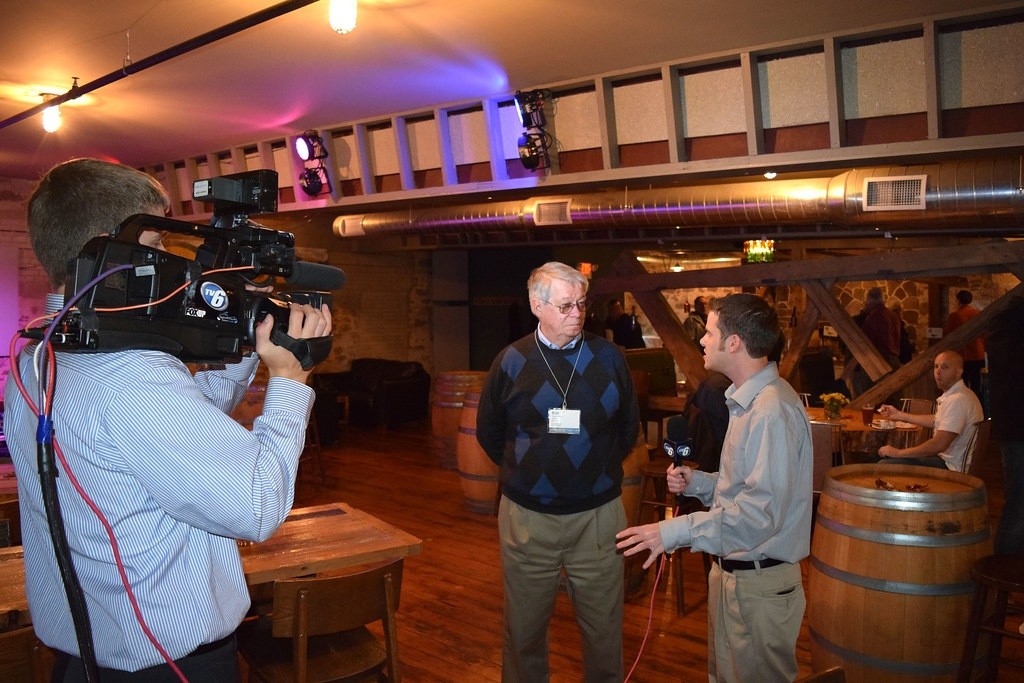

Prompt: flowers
819;392;851;413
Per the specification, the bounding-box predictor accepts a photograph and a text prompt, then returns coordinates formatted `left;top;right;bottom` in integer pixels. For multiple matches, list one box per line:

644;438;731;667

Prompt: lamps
512;91;546;129
295;136;329;161
40;92;61;133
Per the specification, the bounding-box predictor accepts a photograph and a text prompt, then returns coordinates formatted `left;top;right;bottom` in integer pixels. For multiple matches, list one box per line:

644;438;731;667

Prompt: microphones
664;415;694;505
255;260;345;290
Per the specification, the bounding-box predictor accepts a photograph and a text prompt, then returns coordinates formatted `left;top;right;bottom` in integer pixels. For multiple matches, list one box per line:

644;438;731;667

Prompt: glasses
539;296;590;314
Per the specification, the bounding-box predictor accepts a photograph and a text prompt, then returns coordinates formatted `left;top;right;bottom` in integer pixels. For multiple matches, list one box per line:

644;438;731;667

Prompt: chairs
900;398;940;441
0;626;70;683
961;417;992;477
237;557;403;683
809;420;846;496
797;393;811;407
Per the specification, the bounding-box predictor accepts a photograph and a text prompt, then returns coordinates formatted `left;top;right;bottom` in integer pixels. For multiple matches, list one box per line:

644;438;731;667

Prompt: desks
805;406;924;455
305;383;340;449
0;501;422;631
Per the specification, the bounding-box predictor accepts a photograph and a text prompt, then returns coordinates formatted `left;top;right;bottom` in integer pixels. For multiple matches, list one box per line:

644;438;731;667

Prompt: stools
633;408;710;618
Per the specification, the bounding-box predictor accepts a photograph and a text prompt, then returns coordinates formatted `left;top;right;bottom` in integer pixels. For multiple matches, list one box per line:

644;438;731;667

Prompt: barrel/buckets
457;391;501;514
623;422;656;600
432;371;488;472
809;462;996;683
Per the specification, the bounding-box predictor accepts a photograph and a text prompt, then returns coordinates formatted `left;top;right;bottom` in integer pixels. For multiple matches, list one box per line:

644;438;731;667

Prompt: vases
823;402;841;421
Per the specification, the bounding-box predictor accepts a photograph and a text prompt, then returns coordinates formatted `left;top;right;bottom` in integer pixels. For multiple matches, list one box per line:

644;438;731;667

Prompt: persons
604;298;645;349
943;290;986;390
475;262;641;683
877;351;984;474
681;296;709;357
839;286;912;412
615;293;814;683
5;158;333;683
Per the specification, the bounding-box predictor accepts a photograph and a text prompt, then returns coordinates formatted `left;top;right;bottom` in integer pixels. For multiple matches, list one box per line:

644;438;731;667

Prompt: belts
188;634;234;656
711;555;783;572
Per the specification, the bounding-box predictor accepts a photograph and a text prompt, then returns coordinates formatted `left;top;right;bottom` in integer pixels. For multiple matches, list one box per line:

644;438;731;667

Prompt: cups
861;402;874;426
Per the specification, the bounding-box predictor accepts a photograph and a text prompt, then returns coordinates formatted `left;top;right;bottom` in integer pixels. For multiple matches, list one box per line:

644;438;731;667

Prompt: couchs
312;358;430;432
